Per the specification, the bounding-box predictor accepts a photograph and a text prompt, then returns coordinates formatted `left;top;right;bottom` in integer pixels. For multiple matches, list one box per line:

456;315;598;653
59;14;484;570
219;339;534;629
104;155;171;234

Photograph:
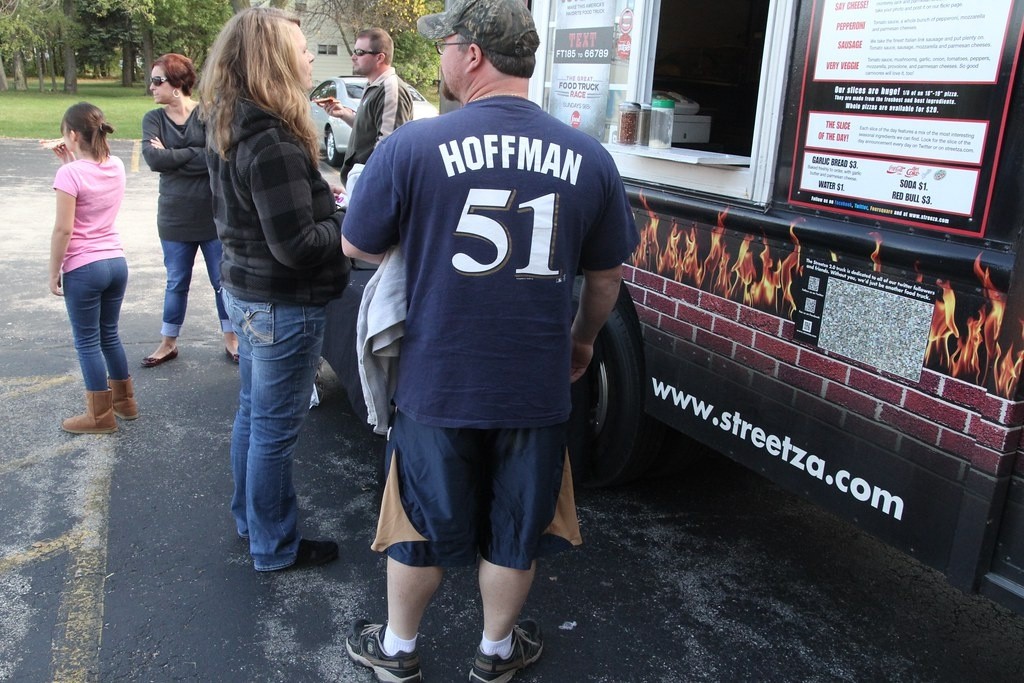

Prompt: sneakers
469;619;543;683
287;538;339;566
346;618;422;683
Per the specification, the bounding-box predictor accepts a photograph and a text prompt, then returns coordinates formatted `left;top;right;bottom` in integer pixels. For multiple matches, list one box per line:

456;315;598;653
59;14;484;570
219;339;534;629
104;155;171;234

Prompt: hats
417;0;536;57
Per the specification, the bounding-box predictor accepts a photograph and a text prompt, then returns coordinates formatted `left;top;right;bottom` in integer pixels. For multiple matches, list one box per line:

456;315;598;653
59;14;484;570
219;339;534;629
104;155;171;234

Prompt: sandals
226;347;239;363
141;345;178;366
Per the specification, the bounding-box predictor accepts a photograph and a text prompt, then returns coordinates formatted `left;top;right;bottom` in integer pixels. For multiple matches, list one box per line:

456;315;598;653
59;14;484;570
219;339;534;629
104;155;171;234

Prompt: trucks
526;1;1024;620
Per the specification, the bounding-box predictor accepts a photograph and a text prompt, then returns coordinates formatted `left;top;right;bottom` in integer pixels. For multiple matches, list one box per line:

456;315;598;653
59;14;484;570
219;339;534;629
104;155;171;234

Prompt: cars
308;76;440;167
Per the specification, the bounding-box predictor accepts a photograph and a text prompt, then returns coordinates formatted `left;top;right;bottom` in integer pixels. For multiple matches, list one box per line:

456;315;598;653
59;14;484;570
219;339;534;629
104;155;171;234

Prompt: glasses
433;40;483;56
354;48;386;57
150;76;167;86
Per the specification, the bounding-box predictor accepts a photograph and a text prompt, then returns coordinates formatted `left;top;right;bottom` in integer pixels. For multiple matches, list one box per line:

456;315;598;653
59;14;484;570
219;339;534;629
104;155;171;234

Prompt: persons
46;100;141;434
191;5;357;576
338;0;641;683
307;23;415;192
141;52;242;368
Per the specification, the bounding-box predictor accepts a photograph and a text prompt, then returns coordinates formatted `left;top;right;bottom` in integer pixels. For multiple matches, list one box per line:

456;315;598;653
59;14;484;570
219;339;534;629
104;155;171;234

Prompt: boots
107;374;139;419
62;386;118;433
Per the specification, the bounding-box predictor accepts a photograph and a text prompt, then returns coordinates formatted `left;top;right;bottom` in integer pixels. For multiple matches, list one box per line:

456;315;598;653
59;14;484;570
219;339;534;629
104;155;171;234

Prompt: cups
648;98;674;149
